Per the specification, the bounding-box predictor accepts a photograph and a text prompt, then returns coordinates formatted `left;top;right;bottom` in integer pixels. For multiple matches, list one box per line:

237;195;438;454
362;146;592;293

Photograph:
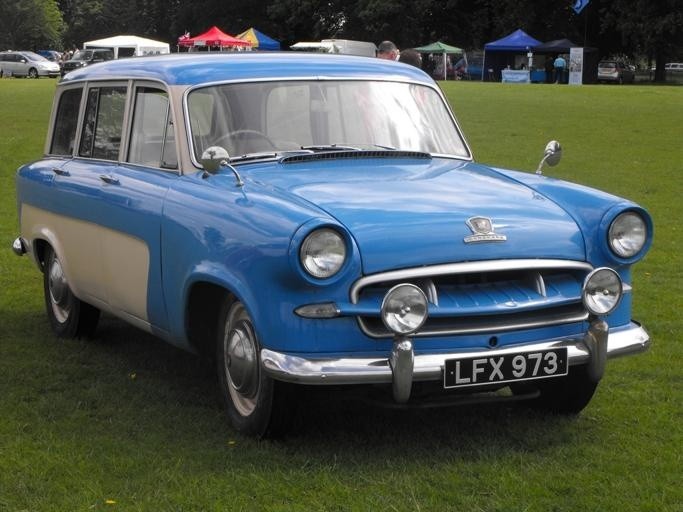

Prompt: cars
10;51;654;440
60;49;113;78
0;50;60;80
32;50;63;69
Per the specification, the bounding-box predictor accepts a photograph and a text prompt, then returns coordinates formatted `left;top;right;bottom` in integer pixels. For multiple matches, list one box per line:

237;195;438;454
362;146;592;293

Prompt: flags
570;0;589;13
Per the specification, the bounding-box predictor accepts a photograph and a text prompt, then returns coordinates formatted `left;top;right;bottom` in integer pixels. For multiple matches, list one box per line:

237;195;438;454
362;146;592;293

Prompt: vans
596;60;635;85
651;62;682;74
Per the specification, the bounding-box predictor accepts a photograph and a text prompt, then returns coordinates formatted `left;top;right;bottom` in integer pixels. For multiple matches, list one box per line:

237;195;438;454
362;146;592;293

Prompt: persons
58;47;81;63
372;40;567;86
